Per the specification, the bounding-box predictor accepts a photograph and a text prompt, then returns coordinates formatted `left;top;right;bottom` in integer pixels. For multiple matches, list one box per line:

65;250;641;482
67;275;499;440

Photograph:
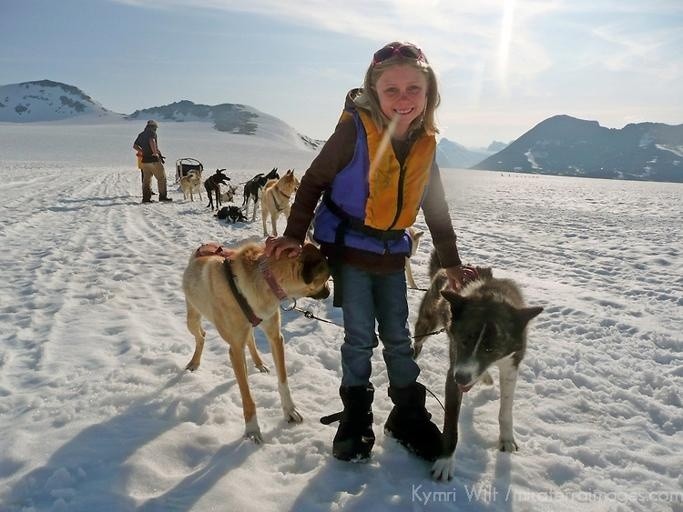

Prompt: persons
136;148;144;183
132;119;173;204
264;40;479;464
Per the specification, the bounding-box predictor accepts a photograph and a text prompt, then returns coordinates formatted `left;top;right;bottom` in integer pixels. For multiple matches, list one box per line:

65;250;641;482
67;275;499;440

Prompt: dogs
260;169;301;238
404;226;425;289
243;168;281;223
213;205;248;226
411;248;546;485
177;170;203;202
219;182;238;204
180;240;332;447
204;168;231;211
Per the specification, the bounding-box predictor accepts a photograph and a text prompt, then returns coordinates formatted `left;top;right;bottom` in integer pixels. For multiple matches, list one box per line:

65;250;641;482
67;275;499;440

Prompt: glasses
372;46;425;65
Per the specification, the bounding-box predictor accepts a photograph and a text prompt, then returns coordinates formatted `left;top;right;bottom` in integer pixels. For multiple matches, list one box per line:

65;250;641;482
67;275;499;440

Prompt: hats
147;120;157;127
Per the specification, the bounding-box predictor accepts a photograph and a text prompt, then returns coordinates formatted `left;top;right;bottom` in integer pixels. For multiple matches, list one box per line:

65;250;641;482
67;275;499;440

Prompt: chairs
174;156;203;185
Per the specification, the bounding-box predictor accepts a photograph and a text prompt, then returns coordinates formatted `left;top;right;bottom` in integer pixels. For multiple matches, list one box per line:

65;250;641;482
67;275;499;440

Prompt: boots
383;383;441;460
333;382;376;464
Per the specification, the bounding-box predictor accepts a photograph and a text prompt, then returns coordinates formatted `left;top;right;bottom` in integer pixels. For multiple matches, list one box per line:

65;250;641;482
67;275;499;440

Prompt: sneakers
159;195;172;201
